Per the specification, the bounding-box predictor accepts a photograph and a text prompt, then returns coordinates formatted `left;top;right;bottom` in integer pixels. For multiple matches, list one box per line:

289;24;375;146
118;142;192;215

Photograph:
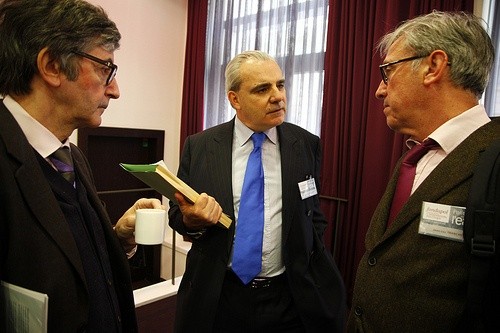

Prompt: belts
228;269;286;289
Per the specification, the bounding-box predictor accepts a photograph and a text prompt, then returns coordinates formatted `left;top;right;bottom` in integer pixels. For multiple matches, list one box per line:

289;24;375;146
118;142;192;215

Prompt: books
120;160;232;228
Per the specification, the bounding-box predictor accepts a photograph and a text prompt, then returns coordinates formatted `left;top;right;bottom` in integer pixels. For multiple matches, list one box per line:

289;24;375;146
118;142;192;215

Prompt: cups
133;209;166;245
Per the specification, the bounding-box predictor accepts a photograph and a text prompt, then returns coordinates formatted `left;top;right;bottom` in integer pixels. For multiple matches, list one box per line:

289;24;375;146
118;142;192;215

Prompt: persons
0;0;166;333
167;51;348;333
348;9;500;333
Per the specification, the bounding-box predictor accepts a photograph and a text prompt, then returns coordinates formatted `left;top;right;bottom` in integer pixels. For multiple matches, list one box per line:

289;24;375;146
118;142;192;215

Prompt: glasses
71;48;117;86
379;52;452;86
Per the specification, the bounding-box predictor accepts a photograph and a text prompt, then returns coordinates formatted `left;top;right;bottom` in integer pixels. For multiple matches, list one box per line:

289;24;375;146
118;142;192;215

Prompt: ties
231;134;266;285
387;138;435;230
47;147;75;187
